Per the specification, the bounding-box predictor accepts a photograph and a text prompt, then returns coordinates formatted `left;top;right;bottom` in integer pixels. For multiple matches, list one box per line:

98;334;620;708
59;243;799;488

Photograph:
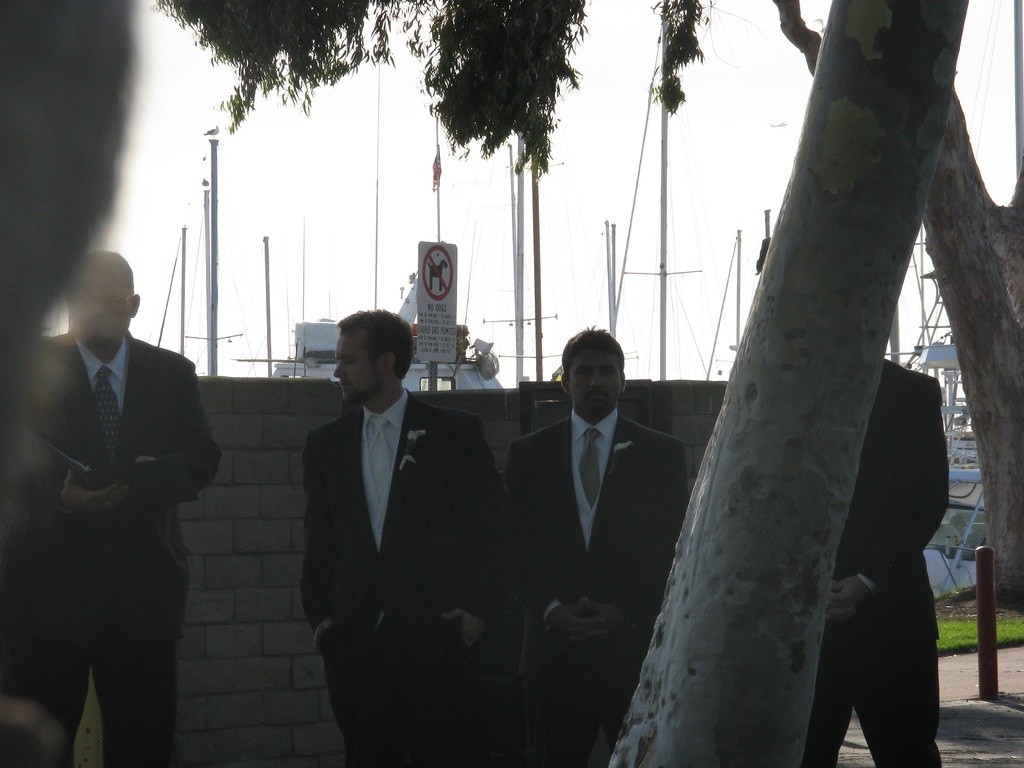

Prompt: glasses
72;293;134;309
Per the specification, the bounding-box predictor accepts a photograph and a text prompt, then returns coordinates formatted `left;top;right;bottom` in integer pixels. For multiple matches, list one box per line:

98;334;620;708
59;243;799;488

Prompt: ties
579;428;601;508
369;416;392;503
94;366;121;464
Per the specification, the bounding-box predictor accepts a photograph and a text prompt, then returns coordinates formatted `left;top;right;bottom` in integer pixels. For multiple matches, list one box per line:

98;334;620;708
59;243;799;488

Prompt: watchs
621;611;635;633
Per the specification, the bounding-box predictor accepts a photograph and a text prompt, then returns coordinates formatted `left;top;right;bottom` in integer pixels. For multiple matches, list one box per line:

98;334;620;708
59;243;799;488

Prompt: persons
505;331;688;768
0;0;223;768
299;311;515;768
800;356;948;767
